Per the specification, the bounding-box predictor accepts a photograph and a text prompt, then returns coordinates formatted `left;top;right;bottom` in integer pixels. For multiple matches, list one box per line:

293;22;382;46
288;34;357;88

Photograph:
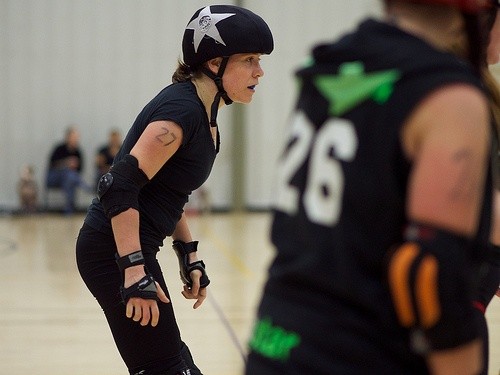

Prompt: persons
246;0;500;375
96;131;123;190
76;5;273;375
46;126;97;215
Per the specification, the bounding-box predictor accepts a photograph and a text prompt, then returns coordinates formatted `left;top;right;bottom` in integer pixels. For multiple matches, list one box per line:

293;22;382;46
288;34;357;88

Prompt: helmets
182;4;275;66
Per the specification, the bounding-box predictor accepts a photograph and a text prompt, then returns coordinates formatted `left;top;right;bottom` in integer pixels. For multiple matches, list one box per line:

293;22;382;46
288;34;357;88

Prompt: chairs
37;156;86;213
